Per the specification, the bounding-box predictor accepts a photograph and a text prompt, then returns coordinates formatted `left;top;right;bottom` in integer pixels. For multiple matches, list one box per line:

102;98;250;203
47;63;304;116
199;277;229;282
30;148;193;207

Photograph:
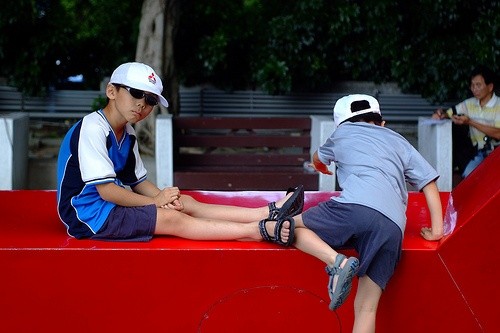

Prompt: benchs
169;113;321;190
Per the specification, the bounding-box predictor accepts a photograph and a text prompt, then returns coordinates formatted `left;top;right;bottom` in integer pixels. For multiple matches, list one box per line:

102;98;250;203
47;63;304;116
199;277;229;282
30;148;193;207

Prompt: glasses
111;83;158;106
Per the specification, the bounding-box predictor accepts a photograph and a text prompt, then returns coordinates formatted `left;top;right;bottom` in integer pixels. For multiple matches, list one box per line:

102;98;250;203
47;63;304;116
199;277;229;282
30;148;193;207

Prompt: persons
292;93;445;333
56;61;305;248
431;63;500;178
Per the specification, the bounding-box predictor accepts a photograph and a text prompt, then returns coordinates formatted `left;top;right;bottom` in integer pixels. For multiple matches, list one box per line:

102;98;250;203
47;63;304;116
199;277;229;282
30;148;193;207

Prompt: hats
108;62;168;107
333;94;381;128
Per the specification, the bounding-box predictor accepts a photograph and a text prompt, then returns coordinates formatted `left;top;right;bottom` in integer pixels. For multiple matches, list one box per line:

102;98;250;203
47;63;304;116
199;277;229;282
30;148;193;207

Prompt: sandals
268;185;304;221
325;252;359;310
258;216;294;246
